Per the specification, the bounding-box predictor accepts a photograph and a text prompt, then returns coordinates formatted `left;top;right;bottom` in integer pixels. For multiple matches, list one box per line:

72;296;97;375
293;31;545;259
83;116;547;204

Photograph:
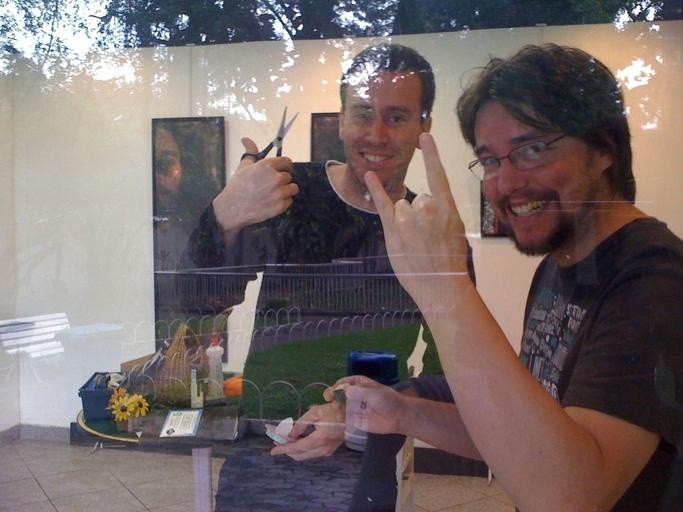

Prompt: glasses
465;130;573;184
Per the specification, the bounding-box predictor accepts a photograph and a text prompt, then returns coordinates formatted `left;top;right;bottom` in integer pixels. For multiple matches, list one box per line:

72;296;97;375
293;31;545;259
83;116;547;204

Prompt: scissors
241;106;300;163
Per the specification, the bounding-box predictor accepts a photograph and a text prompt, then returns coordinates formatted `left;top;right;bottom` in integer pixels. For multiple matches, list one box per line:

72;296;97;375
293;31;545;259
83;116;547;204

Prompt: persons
323;42;683;511
175;44;478;511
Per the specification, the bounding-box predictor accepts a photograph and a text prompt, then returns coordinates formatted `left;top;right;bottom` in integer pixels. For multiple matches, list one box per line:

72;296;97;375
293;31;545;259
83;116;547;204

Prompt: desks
74;371;238;512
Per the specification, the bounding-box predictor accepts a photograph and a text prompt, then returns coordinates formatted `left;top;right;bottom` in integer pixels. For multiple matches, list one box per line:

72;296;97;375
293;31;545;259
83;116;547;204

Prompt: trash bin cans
345;350;401;456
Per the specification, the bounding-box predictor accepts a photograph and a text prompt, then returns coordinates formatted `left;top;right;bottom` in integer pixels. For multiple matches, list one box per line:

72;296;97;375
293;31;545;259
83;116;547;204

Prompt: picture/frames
308;112;346;165
149;115;229;365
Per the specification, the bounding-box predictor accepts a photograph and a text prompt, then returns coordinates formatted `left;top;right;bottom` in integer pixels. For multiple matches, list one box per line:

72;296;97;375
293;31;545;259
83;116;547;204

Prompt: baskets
119;324;210;406
